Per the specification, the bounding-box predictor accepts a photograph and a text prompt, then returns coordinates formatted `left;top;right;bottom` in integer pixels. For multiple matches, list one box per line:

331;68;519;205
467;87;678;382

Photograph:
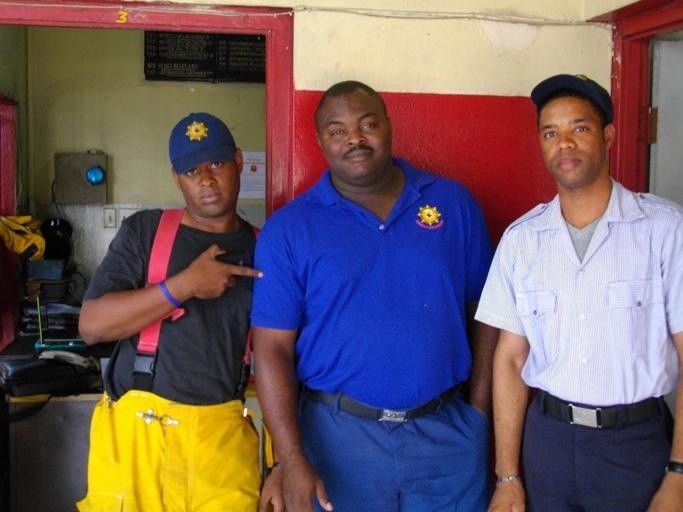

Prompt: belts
542;393;660;431
299;384;461;424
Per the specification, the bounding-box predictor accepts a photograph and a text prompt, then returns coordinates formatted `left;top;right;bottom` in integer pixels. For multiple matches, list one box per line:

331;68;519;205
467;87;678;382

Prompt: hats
170;113;238;173
532;73;614;122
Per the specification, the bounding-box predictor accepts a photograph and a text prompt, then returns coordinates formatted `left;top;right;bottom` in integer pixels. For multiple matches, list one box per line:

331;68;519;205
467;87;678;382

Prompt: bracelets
160;282;183;308
496;476;520;485
667;463;682;475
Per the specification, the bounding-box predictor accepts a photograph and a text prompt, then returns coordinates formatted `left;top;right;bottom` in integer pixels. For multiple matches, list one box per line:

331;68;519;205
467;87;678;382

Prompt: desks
4;384;264;512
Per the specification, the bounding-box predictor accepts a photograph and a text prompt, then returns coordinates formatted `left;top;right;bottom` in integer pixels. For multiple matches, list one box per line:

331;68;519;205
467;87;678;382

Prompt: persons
250;81;500;512
76;111;262;512
474;75;682;512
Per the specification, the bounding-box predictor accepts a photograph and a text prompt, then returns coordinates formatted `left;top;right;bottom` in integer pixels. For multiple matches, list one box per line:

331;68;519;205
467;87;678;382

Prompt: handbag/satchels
0;358;78;397
